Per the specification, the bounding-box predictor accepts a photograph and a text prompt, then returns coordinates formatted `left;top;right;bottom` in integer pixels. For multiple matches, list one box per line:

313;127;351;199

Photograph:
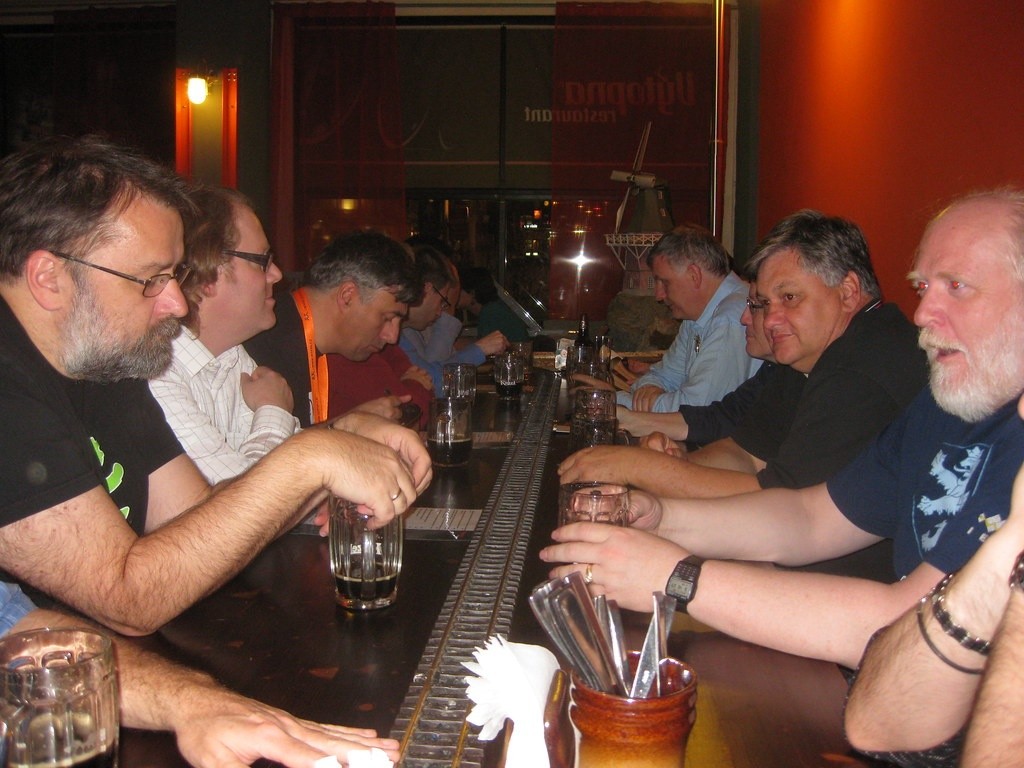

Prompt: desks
119;350;868;767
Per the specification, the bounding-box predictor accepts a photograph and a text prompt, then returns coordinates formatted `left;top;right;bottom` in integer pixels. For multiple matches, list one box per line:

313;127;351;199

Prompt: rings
392;487;401;501
585;563;593;584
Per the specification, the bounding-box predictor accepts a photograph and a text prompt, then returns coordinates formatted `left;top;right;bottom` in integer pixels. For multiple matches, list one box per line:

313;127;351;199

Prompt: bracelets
931;571;992;655
917;588;985;674
1009;550;1024;589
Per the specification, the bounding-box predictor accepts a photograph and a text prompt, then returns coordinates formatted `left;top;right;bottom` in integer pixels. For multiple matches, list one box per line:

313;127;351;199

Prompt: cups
557;480;631;527
443;363;477;402
0;625;121;768
566;348;596;388
427;397;473;468
509;341;534;379
570;410;635;451
593;336;612;373
575;388;619;413
494;354;524;398
569;650;697;768
328;492;405;609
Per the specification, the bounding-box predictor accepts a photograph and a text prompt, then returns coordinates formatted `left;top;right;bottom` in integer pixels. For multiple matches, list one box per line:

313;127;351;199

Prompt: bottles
574;314;594;373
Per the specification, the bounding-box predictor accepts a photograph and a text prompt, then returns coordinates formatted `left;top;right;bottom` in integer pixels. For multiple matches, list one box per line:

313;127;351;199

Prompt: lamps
183;57;215;104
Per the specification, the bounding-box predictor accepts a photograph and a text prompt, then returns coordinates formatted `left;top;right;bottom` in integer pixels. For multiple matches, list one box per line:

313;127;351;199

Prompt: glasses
431;285;452;311
745;295;765;314
219;248;275;273
47;250;193;298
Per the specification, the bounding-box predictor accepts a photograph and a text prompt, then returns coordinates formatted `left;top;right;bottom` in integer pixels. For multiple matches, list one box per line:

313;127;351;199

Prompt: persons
842;395;1024;768
149;189;301;485
400;253;531;393
558;211;929;584
539;186;1024;672
0;581;400;768
242;233;424;426
0;136;434;637
567;222;763;414
583;271;791;442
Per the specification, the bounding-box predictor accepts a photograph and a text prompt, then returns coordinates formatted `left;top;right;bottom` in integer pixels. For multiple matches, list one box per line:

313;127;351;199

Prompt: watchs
665;556;706;614
